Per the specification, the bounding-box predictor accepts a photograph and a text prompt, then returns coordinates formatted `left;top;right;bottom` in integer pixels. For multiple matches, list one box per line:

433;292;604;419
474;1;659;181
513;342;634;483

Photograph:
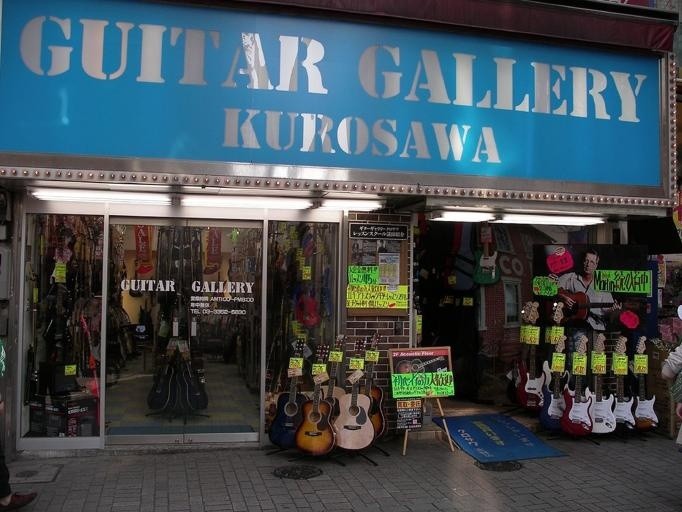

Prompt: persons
0;338;38;512
673;366;682;418
557;247;623;331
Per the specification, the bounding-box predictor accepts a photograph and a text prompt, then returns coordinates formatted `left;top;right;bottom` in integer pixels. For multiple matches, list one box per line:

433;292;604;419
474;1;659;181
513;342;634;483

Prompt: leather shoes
0;492;37;512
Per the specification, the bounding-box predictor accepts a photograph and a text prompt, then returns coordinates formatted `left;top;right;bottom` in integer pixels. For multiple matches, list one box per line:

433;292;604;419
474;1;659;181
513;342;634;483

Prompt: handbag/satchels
669;369;682;402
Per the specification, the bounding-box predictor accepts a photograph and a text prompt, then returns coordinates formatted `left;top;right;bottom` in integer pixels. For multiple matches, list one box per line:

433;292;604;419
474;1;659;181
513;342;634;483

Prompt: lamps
27;187;176;207
425;210;501;222
487;213;609;226
319;200;385;212
178;194;318;211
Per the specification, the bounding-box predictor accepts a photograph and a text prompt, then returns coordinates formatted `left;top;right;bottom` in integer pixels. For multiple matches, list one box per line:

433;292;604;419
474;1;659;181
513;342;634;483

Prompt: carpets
431;413;570;464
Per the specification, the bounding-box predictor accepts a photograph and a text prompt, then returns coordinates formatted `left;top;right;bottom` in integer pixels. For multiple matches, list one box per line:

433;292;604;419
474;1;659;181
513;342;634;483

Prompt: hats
677;305;682;320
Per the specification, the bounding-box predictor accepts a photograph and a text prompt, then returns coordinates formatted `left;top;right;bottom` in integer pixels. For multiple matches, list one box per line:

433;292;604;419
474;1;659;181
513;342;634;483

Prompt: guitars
146;350;178;412
612;336;635;431
563;335;593;435
551;291;623;322
473;224;500;284
542;303;570;393
180;355;209;411
520;303;545;407
635;335;659;428
321;334;346;406
507;301;533;404
395;356;445;373
294;344;337;455
585;333;616;433
547;335;566;428
359;333;388;439
268;338;306;448
333;339;376;450
